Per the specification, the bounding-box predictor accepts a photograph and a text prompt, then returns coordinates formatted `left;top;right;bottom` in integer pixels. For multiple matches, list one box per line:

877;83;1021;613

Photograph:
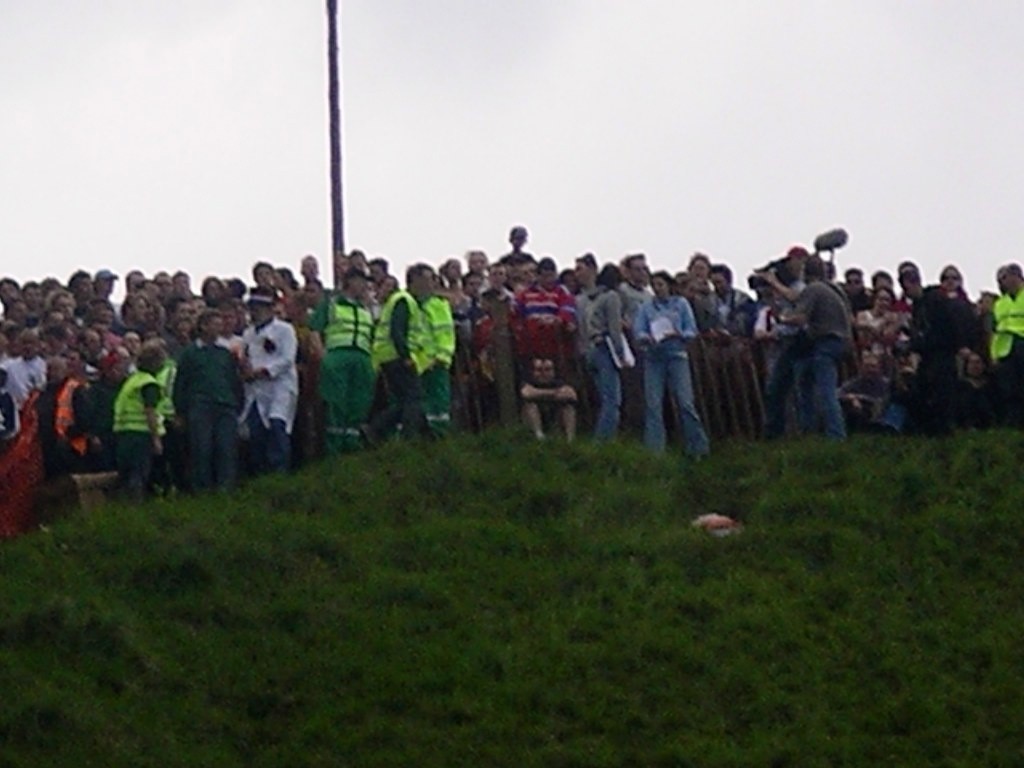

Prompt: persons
776;254;853;442
173;309;247;492
113;343;168;501
497;228;541;294
355;264;440;450
0;247;1024;549
634;270;712;462
517;358;585;444
580;262;634;441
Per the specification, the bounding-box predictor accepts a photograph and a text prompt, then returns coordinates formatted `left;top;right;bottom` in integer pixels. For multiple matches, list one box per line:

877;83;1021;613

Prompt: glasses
943;276;958;281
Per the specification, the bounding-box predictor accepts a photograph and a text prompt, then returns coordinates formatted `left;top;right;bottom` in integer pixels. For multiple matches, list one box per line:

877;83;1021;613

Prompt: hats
245;287;273;305
346;268;373;281
790;247;809;260
94;270;118;280
